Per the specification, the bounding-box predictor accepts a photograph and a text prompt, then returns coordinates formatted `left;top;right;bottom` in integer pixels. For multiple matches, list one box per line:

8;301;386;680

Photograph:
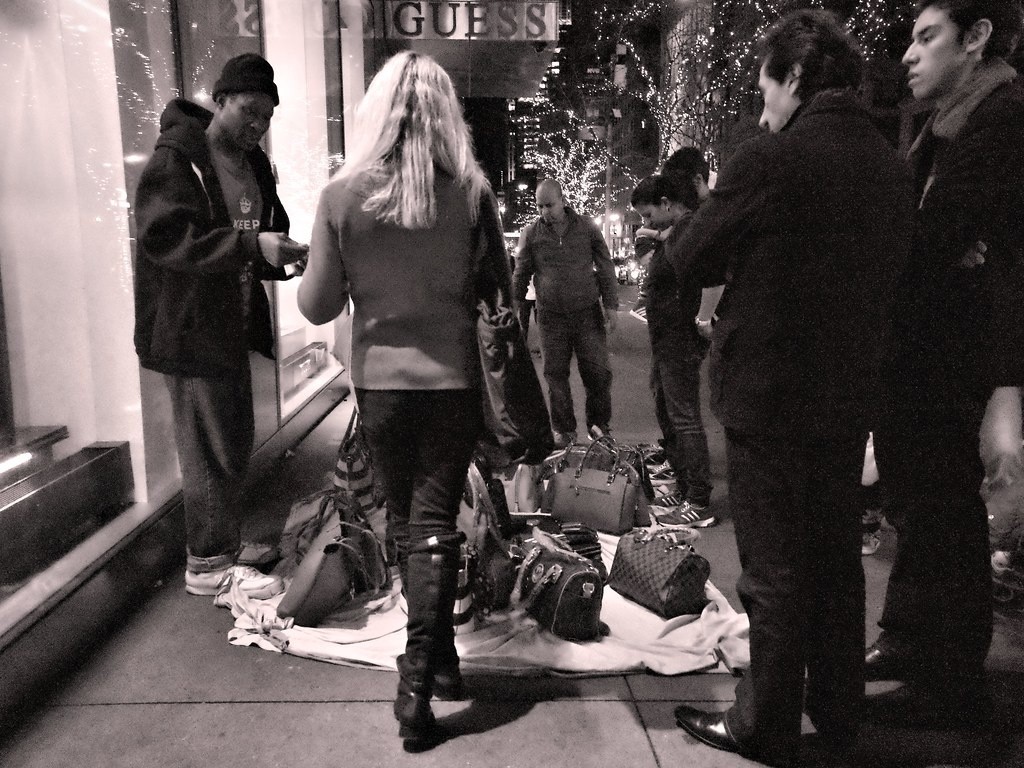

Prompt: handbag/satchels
276;408;392;627
609;529;711;619
468;521;610;645
469;436;652;534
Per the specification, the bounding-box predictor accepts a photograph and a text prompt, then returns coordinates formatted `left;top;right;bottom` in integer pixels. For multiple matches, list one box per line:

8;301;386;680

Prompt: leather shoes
864;632;904;682
866;682;996;729
673;704;733;752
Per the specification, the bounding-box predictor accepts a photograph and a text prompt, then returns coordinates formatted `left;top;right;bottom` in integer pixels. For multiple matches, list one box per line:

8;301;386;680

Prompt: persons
133;53;309;601
632;1;1023;767
297;52;513;739
512;178;619;449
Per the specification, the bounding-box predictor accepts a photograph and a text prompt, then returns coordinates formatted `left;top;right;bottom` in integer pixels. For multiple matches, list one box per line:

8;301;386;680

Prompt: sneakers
654;491;684;509
644;451;667;470
234;540;279;564
657;499;716;530
629;300;647;321
184;566;284;600
649;469;677;485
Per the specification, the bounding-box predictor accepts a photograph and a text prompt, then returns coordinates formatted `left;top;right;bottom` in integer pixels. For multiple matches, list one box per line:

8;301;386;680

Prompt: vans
503;231;520;259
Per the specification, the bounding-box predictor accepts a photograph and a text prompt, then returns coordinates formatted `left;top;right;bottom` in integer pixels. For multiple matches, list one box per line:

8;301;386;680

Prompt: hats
213;53;279;105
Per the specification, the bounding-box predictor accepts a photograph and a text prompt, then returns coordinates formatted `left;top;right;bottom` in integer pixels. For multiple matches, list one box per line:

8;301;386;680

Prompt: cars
608;248;650;287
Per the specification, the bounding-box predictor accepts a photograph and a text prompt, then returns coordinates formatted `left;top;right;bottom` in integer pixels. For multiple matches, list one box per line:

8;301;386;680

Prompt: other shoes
586;426;616;452
554;431;578;448
861;532;880;556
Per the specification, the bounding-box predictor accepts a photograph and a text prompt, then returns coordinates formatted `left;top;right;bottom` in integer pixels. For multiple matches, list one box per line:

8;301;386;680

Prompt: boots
396;536;464;703
393;531;466;741
